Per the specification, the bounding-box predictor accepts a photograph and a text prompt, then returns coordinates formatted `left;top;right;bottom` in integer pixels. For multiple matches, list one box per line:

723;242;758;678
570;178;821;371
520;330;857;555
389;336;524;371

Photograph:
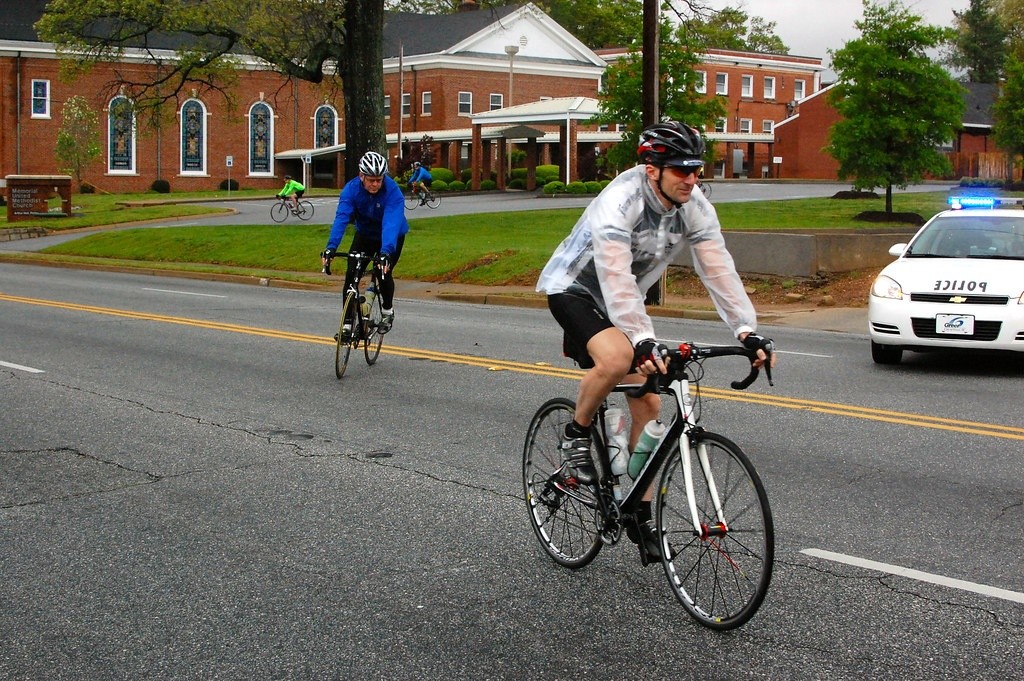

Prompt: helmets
637;121;705;167
358;152;388;177
415;162;421;166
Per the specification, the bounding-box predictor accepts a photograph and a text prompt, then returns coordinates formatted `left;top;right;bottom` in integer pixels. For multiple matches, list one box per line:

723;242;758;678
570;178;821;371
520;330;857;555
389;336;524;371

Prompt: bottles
364;287;375;314
603;403;630;476
627;418;665;480
359;296;368;316
289;203;294;212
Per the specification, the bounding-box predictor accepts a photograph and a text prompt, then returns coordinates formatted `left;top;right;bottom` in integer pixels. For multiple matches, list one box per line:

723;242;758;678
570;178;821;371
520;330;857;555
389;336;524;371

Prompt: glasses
672;166;705;178
366;177;381;182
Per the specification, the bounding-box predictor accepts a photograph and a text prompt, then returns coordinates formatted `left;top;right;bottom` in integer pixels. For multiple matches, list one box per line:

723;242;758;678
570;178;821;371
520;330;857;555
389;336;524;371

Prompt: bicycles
518;329;776;633
319;251;395;379
271;196;313;222
696;181;712;199
403;182;442;210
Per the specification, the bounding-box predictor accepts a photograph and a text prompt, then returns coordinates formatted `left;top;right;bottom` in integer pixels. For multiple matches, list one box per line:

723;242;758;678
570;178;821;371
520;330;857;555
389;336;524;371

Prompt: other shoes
289;203;294;208
431;196;435;201
420;202;425;206
293;210;299;215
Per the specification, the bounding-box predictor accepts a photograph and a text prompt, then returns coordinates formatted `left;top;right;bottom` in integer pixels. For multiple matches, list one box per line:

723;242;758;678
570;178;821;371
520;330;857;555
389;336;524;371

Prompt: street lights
505;46;520;183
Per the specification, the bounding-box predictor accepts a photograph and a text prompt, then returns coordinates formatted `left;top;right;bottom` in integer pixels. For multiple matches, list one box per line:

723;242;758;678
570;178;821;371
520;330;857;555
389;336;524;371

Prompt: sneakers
334;328;351;342
377;309;394;334
626;520;676;558
561;424;599;485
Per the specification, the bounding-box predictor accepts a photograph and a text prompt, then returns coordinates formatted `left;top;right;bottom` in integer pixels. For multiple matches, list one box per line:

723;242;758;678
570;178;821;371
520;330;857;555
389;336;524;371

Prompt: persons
535;120;775;561
322;152;409;339
276;176;305;214
408;162;433;206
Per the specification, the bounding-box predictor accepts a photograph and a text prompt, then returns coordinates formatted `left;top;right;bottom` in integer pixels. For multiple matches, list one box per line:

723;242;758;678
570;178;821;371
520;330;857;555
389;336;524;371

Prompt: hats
285;175;292;179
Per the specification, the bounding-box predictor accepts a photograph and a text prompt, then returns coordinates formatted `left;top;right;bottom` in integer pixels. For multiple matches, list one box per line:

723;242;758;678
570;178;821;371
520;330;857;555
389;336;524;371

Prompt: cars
866;196;1024;371
